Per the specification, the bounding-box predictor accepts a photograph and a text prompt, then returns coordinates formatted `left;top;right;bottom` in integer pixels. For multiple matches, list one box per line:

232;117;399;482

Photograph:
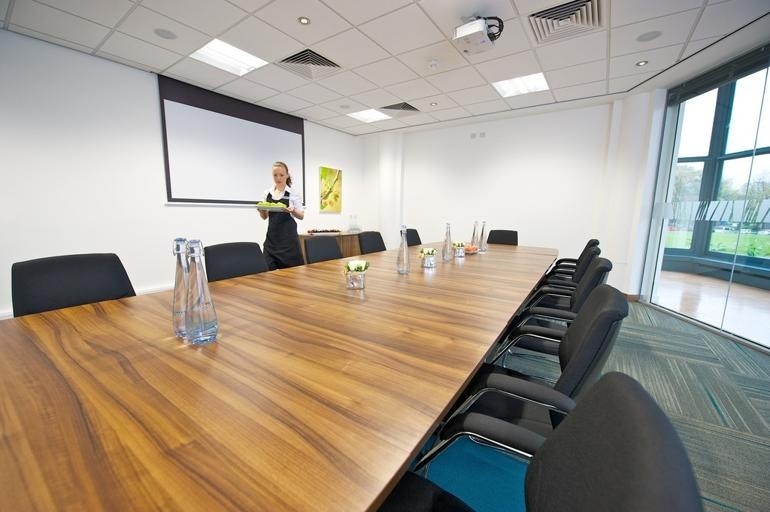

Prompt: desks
1;241;561;512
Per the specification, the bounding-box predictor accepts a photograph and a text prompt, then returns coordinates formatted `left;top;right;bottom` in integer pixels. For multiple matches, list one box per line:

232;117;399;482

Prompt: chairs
11;251;136;318
489;237;613;364
372;470;478;511
432;283;629;445
486;230;519;247
203;243;270;289
304;236;344;265
400;229;423;247
403;371;706;511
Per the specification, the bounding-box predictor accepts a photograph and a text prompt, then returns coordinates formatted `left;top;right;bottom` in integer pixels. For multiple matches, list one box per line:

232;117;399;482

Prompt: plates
254;204;284;213
306;232;341;236
465;248;480;255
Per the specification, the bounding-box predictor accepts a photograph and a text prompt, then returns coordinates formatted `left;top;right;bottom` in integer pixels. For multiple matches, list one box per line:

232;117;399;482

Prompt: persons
257;161;305;268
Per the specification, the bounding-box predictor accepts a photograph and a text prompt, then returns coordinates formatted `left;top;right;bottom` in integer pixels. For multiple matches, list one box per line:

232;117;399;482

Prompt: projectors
454;19;495;56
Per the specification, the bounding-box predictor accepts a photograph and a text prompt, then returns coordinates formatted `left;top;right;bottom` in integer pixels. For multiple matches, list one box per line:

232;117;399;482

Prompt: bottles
346;215;361;234
470;220;479;246
441;223;452;263
396;224;409;274
478;220;489;253
172;236;192;341
184;238;219;345
